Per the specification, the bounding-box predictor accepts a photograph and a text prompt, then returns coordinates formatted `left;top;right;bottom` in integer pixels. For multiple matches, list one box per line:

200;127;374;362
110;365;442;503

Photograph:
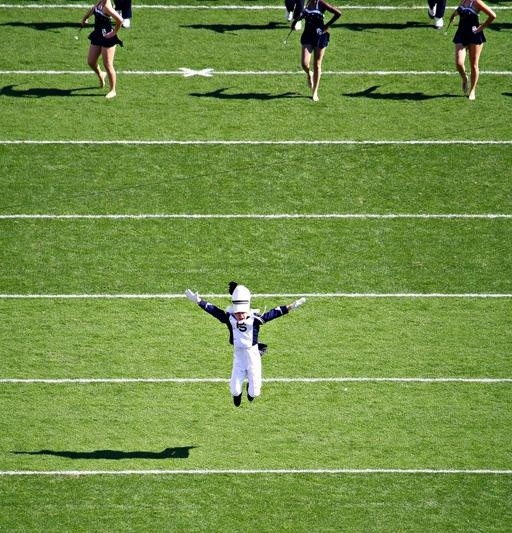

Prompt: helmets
229;282;251;313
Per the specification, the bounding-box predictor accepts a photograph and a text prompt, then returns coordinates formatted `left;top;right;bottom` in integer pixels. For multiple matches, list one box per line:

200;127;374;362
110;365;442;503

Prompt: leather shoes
234;383;254;407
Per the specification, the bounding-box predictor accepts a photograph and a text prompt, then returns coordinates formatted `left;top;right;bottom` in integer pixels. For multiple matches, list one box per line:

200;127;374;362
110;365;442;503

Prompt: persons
427;0;448;29
79;0;122;98
449;0;498;99
185;279;306;406
284;0;306;30
290;0;342;101
111;0;132;28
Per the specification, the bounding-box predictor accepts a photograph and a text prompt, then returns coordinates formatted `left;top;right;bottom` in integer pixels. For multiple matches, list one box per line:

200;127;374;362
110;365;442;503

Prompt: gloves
184;288;201;303
289;297;305;310
